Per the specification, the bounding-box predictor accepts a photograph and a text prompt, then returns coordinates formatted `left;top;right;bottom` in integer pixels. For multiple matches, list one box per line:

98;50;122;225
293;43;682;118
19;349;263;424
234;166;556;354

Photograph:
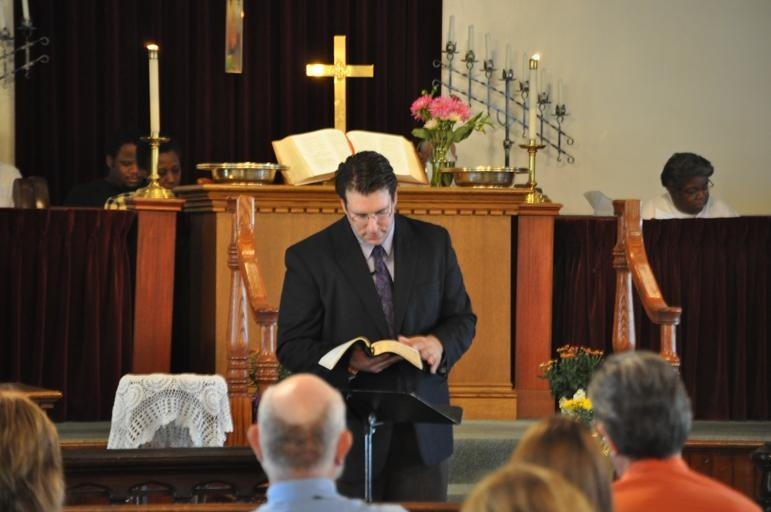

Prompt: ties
370;245;393;340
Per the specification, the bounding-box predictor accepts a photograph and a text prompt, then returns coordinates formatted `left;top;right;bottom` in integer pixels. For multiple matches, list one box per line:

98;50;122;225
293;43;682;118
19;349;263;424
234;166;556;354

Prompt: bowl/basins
194;160;288;183
439;166;529;186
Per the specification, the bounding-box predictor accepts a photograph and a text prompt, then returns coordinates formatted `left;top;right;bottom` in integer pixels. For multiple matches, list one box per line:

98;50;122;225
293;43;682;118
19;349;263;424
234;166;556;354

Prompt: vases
431;139;454;186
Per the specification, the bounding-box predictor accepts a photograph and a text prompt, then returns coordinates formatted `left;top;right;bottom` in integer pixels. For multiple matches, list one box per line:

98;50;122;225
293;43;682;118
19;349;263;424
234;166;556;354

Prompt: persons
276;151;478;504
248;372;408;511
510;413;614;511
459;461;595;512
63;139;148;206
0;391;66;511
135;137;184;199
588;351;763;511
642;151;738;222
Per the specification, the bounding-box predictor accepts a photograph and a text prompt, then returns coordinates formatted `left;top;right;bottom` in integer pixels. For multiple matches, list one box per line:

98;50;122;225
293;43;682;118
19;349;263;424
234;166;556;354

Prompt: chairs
106;371;232;450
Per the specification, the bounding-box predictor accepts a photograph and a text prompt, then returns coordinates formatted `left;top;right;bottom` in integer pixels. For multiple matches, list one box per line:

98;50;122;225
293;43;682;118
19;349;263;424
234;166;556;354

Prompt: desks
553;213;771;423
0;206;132;424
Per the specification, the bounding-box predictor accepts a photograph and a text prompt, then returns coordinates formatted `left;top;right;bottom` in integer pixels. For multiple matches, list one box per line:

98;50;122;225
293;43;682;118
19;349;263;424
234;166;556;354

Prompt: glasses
345;197;393;222
681;178;713;196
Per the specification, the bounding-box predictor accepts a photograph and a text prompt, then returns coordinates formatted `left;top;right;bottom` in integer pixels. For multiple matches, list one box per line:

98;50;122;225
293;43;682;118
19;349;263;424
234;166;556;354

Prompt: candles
22;0;31;26
446;12;564;108
146;42;159;138
0;0;6;32
529;53;539;147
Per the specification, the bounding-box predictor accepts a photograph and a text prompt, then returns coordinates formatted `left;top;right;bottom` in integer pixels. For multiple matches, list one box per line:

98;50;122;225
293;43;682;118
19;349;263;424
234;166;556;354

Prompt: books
317;334;425;376
270;126;431;190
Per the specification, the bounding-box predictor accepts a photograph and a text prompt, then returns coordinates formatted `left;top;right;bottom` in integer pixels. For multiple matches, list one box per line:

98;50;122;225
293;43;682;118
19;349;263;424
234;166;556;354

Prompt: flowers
411;84;494;184
559;397;597;428
540;343;604;396
